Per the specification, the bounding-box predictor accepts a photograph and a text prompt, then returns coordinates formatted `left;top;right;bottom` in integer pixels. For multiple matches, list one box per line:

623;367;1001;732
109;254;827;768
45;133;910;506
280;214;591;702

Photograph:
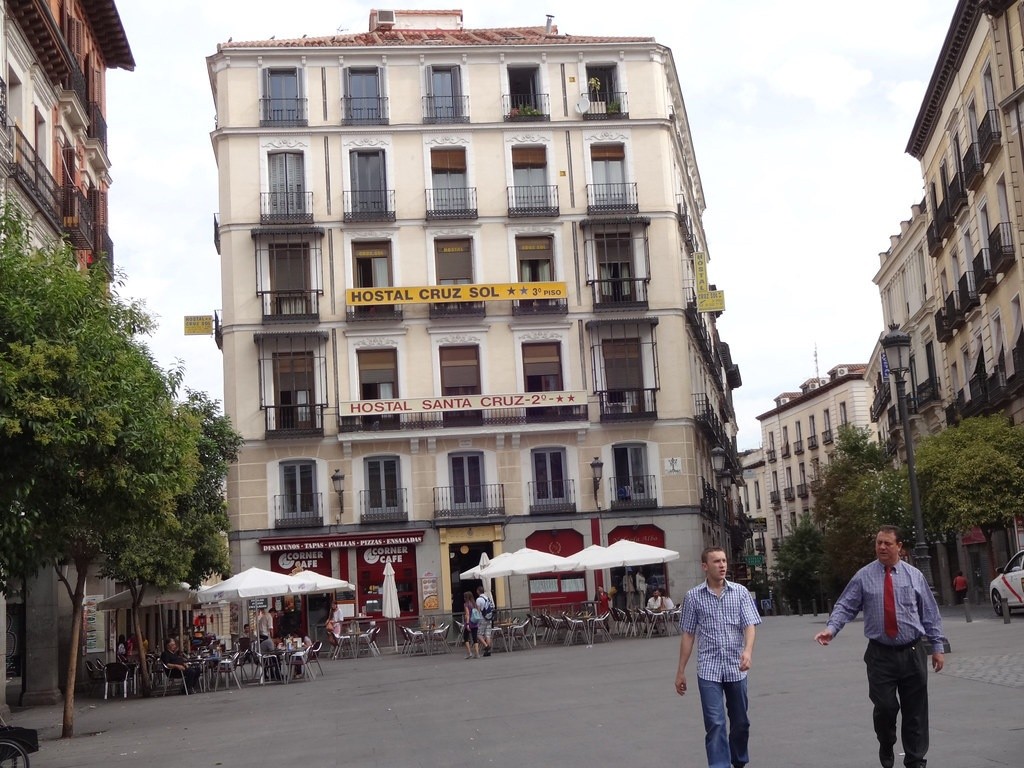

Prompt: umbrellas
197;565;356;686
382;556;400;653
479;552;495;609
95;580;213;653
460;539;679;637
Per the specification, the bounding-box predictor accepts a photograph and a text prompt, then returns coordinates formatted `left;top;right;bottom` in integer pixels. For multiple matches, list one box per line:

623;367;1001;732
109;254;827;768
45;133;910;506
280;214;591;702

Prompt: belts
872;638;920;650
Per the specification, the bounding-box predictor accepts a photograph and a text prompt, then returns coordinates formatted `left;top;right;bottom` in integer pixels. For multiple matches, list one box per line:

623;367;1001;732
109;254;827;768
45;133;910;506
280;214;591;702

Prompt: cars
990;550;1024;615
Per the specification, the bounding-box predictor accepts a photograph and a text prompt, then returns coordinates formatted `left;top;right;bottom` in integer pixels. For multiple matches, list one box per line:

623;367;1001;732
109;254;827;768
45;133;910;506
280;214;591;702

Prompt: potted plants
505;105;550;122
583;101;628;121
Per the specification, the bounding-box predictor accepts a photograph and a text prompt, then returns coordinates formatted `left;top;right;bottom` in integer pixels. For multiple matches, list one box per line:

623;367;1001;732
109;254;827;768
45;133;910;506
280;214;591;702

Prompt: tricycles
0;722;39;768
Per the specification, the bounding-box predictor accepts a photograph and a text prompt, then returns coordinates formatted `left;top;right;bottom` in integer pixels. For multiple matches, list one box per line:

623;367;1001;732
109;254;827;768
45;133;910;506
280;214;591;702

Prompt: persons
238;624;255;642
594;585;612;634
813;524;945;768
645;590;664;634
160;638;201;694
954;567;988;606
324;601;344;659
624;567;637;610
117;630;148;665
675;545;762;768
259;607;277;641
636;570;647;609
176;626;217;652
463;587;495;659
661;589;677;610
304;636;312;647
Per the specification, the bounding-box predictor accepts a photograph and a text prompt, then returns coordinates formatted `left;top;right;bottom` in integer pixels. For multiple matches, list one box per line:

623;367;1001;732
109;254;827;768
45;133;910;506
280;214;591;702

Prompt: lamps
590;456;605;494
331;468;346;513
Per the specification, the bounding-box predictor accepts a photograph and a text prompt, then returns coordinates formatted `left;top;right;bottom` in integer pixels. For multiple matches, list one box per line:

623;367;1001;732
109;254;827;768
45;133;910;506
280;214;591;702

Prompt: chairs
84;604;682;700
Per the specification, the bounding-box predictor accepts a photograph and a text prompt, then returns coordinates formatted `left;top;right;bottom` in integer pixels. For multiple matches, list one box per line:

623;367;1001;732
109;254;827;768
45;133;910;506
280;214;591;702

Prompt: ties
884;566;898;639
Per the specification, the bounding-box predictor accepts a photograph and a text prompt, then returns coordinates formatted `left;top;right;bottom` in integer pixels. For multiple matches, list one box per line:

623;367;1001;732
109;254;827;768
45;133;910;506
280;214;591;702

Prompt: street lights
878;321;937;593
708;443;732;573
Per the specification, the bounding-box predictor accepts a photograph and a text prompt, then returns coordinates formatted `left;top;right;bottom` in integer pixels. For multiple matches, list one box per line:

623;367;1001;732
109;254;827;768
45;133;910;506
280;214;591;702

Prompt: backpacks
479;596;493;620
471;608;480;623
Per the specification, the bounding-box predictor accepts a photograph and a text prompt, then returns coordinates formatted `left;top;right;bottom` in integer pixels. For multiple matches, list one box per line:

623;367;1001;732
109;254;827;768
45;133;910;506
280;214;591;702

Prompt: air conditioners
809;368;848;391
780;398;789;405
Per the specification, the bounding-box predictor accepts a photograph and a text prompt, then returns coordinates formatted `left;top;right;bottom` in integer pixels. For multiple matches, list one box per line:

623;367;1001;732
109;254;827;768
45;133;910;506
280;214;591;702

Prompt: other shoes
904;757;926;768
879;744;894;768
